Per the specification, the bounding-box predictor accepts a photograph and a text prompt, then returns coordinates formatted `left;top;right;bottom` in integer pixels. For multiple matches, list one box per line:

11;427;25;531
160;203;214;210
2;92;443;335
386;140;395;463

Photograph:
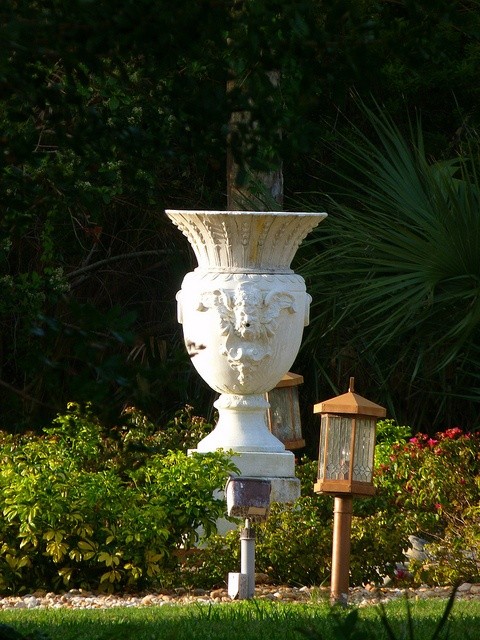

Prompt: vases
163;209;328;451
